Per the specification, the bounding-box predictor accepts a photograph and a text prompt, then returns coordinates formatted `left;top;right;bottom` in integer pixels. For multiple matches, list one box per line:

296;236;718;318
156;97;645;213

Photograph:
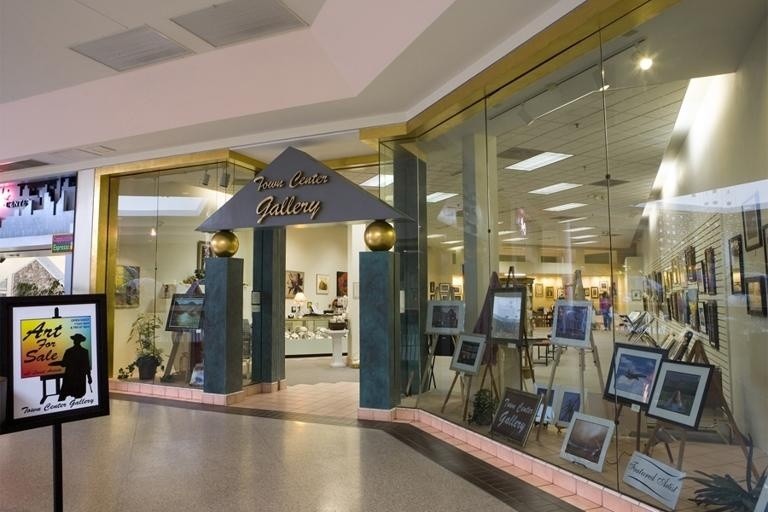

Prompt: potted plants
118;311;166;382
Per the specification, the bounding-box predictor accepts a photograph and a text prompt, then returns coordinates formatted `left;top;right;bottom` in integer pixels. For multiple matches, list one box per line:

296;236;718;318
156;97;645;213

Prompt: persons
666;390;683;411
59;334;92;401
600;292;609;330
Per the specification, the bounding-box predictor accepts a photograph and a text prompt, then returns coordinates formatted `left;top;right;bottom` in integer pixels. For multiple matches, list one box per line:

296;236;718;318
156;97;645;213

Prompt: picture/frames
531;382;559;423
554;384;587;429
727;194;768;321
448;332;487;376
645;358;715;430
113;264;141;308
630;245;719;358
426;300;465;334
535;281;611;300
314;273;332;294
285;269;305;300
485;285;527;344
429;279;463;299
165;291;207;335
558;411;616;474
195;240;213;279
550;297;592;350
601;342;667;409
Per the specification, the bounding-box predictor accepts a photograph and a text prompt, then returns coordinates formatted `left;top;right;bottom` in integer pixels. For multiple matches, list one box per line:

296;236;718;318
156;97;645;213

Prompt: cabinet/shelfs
280;314;350;359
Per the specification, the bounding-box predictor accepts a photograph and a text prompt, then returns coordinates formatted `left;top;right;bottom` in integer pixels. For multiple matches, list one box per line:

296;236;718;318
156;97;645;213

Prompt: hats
70;333;86;341
